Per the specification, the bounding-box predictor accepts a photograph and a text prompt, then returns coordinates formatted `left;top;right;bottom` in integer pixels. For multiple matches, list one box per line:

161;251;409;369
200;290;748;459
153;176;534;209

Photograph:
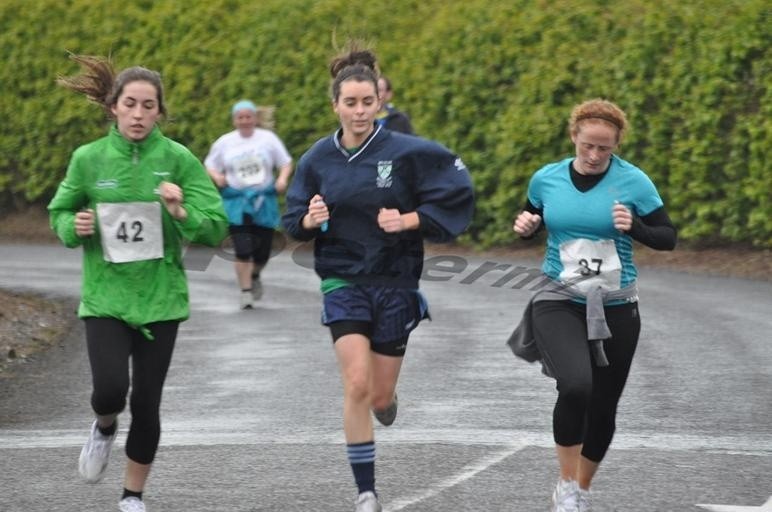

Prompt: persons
506;98;678;511
287;17;473;512
370;75;421;137
204;98;294;312
54;46;234;511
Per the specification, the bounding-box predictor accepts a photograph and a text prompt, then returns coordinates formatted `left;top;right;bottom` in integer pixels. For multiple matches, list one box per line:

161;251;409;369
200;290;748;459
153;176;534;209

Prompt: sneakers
354;491;383;511
242;277;262;308
551;480;593;512
119;497;147;512
77;421;118;482
374;401;398;426
